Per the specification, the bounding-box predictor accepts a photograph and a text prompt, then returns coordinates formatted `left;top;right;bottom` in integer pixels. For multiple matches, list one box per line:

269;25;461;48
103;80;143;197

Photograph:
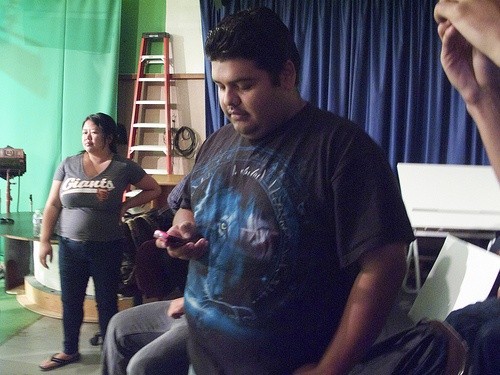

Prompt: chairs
416;317;471;375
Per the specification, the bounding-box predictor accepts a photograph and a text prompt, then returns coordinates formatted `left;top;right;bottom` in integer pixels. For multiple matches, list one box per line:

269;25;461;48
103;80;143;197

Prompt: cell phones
153;230;197;248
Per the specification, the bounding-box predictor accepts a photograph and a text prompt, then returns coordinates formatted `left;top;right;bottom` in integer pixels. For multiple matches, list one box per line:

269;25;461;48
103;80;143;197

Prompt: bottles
32;209;43;237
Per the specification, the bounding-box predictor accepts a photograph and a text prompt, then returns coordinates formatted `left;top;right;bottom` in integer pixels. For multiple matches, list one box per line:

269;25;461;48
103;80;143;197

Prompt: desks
0;212;135;323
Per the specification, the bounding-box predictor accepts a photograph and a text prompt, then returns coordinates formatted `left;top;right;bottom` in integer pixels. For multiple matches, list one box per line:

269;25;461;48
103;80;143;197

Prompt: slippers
39;353;81;370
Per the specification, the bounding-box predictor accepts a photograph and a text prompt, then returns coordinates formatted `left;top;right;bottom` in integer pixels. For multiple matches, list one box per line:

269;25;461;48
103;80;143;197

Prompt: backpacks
348;325;448;375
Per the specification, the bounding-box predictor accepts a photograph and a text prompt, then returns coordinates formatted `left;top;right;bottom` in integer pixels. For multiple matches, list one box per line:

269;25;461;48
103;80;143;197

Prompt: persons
102;296;186;375
39;112;162;371
431;0;500;375
155;8;418;375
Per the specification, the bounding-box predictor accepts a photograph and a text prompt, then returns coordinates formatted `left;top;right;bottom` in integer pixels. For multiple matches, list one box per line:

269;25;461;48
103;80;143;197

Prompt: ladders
126;32;173;177
